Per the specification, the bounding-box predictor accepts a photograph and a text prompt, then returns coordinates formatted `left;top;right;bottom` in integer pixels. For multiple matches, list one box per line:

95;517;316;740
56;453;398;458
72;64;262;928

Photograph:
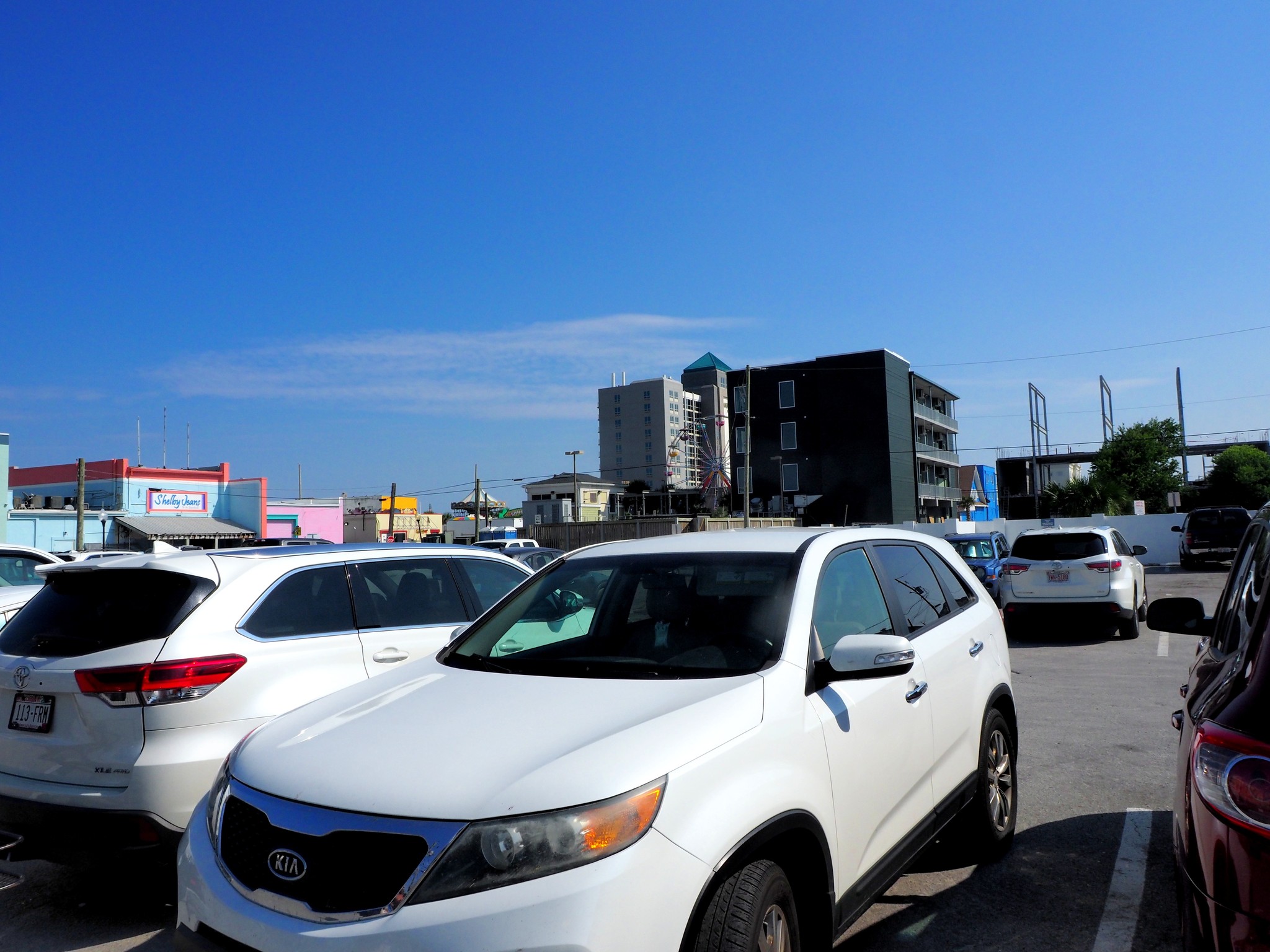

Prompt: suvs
0;543;597;860
168;525;1019;952
1170;500;1270;952
937;531;1010;603
1171;506;1252;570
996;526;1149;639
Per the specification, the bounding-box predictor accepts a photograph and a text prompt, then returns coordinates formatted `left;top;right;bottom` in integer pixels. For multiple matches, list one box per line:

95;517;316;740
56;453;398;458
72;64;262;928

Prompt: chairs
629;578;696;663
391;572;433;626
956;544;965;557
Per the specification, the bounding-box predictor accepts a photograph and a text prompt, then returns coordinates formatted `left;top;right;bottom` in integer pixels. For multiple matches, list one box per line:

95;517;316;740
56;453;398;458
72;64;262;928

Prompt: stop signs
387;536;394;543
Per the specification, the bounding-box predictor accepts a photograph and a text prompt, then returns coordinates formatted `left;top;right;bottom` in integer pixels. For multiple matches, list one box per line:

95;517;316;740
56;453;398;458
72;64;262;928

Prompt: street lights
488;514;492;529
566;450;584;521
98;507;108;550
414;510;422;543
744;363;761;529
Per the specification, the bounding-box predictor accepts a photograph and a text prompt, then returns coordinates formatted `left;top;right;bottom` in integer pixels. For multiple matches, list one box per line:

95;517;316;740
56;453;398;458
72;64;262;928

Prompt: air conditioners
936;432;942;441
935;398;942;407
918;389;925;399
922;462;928;472
920;426;926;435
939;466;945;476
